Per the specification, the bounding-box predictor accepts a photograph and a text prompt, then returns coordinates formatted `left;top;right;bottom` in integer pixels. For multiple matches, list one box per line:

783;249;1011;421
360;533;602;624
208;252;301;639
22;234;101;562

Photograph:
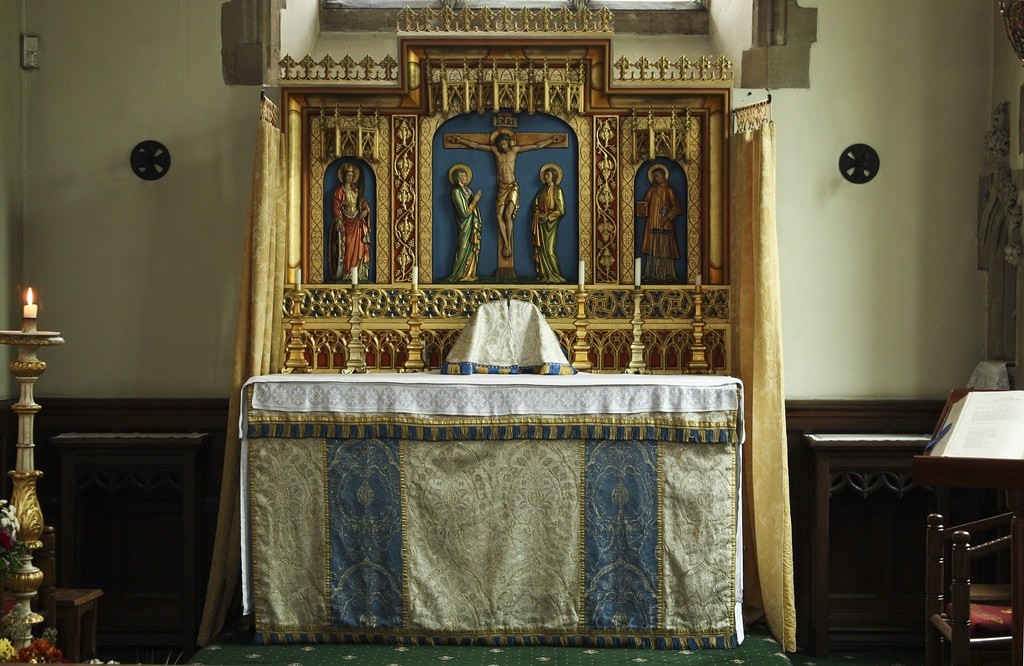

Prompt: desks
53;431;211;650
802;431;987;653
236;368;748;650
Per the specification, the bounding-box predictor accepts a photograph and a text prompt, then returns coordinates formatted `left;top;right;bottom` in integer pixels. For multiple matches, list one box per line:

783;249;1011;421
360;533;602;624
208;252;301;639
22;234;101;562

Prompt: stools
41;586;104;663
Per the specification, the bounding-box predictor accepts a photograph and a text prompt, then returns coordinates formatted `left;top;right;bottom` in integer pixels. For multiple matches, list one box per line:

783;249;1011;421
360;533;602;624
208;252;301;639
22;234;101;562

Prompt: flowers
0;499;70;666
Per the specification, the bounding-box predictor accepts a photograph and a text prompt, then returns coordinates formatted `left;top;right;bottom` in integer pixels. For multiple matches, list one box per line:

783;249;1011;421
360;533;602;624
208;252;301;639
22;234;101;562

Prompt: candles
23;288;37;319
635;258;641;287
411;267;418;284
351;267;358;285
295;268;301;284
578;260;584;285
696;274;702;285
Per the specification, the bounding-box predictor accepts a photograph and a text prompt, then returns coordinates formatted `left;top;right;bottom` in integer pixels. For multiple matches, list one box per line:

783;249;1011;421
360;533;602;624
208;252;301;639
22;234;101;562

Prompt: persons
642;164;681;281
447;134;566;257
333;162;370;280
446;163;482;282
530;162;565;282
